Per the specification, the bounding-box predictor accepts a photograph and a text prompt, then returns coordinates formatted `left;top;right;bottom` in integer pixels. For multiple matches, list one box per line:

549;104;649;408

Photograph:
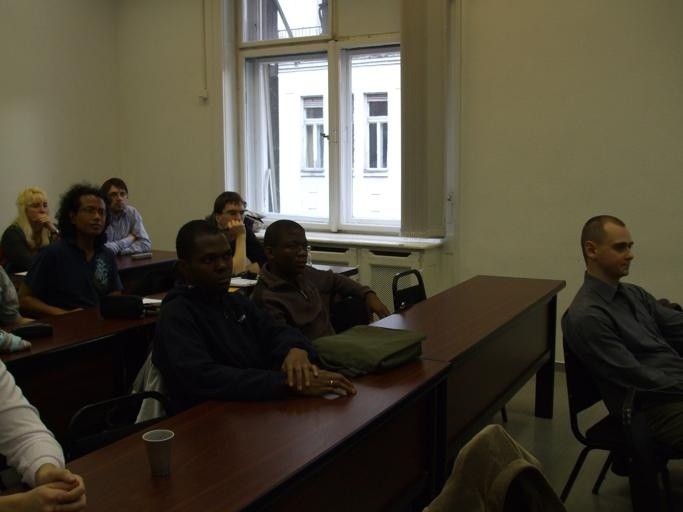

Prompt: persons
149;219;358;401
99;177;152;257
248;218;391;342
18;182;124;315
1;266;36;353
0;185;61;273
204;191;268;276
0;359;88;512
564;215;683;461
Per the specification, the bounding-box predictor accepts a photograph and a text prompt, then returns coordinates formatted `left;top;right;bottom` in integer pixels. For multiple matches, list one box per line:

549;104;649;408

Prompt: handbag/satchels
311;325;427;378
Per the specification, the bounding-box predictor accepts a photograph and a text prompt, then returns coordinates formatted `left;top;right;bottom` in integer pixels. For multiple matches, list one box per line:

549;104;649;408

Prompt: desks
0;360;449;512
369;274;567;488
8;250;180;296
0;265;359;366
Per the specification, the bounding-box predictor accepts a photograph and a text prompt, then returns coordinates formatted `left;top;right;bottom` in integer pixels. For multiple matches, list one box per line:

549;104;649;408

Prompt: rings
329;380;333;386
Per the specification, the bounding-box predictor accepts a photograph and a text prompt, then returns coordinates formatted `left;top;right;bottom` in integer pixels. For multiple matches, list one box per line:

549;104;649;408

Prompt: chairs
393;269;509;421
559;308;623;501
62;391;176;463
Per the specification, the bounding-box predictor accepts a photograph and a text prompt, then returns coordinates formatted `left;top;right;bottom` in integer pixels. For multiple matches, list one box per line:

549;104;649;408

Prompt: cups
142;429;175;481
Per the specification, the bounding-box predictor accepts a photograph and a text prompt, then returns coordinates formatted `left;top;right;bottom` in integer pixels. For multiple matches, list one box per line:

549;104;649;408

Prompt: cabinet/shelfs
254;229;444;323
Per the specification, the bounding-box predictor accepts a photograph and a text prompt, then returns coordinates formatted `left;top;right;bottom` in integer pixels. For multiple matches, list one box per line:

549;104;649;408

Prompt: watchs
50;229;59;236
